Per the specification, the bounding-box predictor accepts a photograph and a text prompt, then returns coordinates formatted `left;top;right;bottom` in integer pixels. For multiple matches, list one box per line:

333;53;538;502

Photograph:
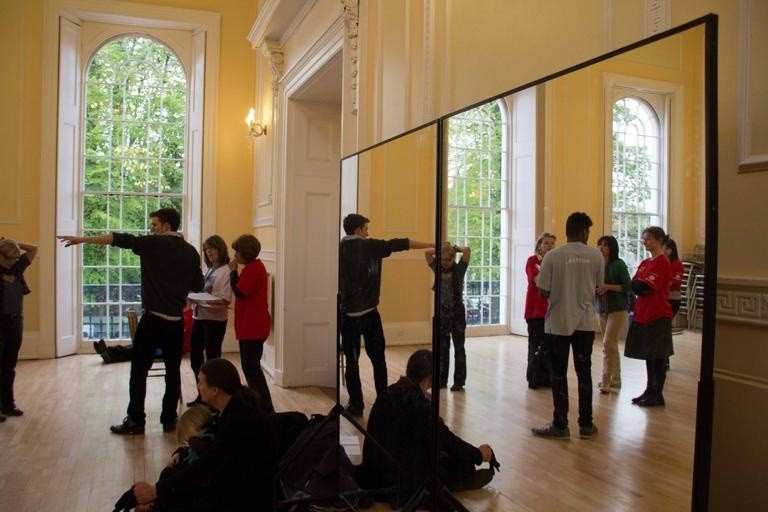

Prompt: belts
7;314;17;319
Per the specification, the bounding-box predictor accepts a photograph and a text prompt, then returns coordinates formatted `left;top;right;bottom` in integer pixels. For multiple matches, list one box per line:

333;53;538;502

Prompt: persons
530;213;604;442
663;241;685;317
623;226;673;408
363;345;500;495
186;234;232;404
524;234;555;389
127;357;292;512
0;236;38;423
422;241;472;390
93;295;193;363
156;406;288;512
339;214;436;418
593;234;631;395
56;207;204;434
228;234;275;412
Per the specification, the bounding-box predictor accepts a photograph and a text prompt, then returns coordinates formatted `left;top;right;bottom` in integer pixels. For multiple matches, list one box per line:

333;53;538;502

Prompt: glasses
1;252;20;262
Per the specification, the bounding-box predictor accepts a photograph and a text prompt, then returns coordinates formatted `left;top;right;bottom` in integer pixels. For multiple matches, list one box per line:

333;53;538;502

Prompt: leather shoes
162;417;177;433
344;401;362;417
93;340;100;354
109;423;145;434
638;391;665;406
448;470;491;492
450;384;461;391
97;339;111;362
1;407;23;416
631;389;648;403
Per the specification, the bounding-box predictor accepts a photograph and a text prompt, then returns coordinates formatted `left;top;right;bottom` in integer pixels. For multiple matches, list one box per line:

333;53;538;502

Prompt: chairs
676;243;704;332
127;305;183;406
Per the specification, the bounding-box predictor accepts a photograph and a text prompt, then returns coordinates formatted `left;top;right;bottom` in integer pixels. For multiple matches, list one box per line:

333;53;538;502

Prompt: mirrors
435;13;720;511
335;118;440;436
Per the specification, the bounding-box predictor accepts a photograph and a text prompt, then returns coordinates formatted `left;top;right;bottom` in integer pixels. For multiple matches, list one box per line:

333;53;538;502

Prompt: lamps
245;107;267;136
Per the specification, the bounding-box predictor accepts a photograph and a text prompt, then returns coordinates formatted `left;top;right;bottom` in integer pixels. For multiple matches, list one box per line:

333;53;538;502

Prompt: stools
338;343;347;386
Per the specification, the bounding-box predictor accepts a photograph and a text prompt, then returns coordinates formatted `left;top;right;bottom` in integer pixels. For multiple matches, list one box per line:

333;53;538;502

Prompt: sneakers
598;380;620;388
579;424;597;438
531;424;570;440
599;381;608;394
